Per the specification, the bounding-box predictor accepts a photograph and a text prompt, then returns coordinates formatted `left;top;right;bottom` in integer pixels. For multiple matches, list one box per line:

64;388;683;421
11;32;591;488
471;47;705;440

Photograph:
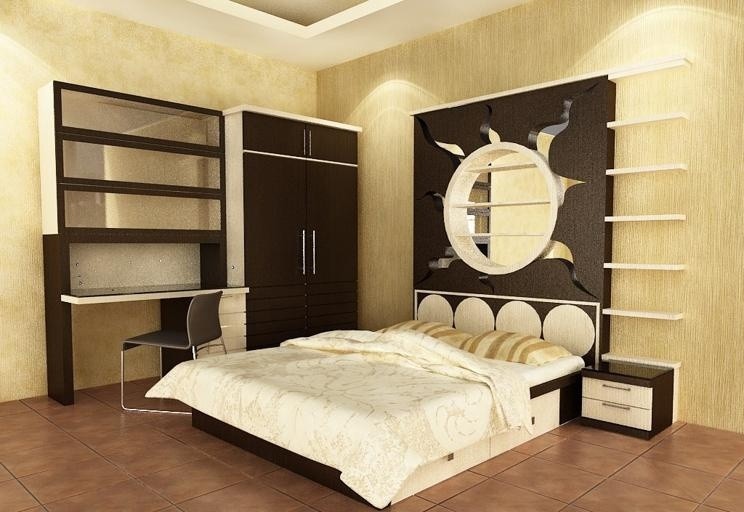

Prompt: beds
145;321;583;511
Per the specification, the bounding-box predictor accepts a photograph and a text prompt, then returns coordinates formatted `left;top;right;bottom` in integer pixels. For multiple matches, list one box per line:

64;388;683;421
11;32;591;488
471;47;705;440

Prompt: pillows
375;319;469;352
463;330;572;366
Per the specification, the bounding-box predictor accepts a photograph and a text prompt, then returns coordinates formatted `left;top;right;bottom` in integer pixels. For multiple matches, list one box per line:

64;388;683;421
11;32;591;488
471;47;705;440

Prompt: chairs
120;291;228;415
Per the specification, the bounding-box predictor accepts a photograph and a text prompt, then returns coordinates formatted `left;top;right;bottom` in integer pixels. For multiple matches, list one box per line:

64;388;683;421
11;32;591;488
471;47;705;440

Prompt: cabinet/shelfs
454;162;550;237
39;80;249;408
225;104;364;350
599;55;694;370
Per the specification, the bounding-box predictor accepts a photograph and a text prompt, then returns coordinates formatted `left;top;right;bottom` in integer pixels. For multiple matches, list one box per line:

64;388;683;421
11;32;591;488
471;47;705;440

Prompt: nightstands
579;362;674;440
